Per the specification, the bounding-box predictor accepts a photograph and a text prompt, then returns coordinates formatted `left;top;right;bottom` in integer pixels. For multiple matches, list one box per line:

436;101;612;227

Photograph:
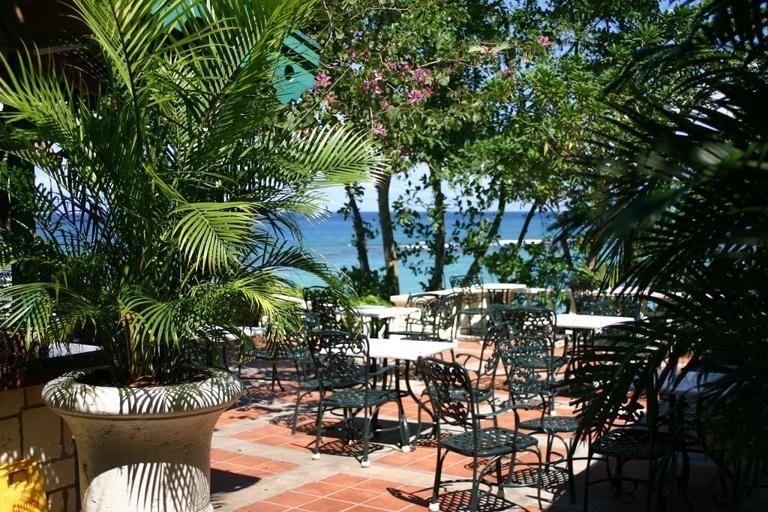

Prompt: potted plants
0;0;394;511
